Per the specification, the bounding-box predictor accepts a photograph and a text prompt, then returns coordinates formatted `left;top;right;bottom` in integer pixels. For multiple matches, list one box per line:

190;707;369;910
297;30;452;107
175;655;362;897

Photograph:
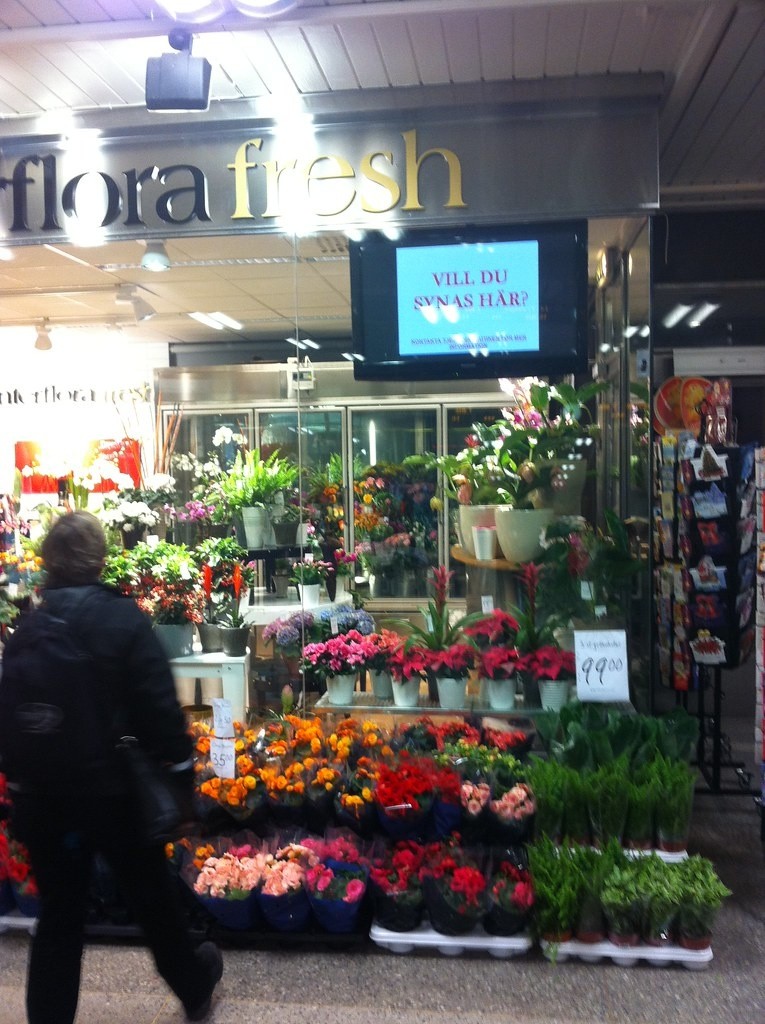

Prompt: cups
472;527;496;561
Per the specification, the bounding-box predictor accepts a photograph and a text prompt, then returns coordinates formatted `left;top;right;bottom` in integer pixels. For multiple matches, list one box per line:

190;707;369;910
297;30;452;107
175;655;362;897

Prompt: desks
252;660;326;718
315;691;545;728
239;545;339;607
168;642;251;729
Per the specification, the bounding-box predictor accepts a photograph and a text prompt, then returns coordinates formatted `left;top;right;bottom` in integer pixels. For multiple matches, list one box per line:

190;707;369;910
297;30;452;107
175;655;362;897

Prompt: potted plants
529;707;734;949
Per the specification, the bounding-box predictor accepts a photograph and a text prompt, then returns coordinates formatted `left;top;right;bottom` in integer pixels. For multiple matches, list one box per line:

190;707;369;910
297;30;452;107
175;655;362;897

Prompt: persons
0;510;224;1024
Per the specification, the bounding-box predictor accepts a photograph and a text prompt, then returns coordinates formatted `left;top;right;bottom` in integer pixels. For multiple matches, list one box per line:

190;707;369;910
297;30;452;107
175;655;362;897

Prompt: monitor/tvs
349;218;589;381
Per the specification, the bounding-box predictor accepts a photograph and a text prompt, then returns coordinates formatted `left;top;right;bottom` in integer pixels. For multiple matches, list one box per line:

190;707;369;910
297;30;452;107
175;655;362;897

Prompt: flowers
1;376;610;919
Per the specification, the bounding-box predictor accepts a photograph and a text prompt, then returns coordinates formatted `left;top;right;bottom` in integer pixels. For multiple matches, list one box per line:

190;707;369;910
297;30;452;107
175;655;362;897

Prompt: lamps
144;31;214;110
114;293;155;321
140;238;171;272
35;321;53;351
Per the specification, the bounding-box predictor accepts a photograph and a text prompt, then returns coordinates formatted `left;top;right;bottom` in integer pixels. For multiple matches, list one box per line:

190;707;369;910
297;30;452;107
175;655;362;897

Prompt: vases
234;507;273;548
272;574;290;598
528;459;587;516
270;521;302;545
204;522;229;542
324;675;357;705
153;622;196;661
457;506;509;555
390;676;420;707
392;569;411;598
297;581;320;607
121;527;145;552
538;679;570;712
493;507;557;562
196;621;222;653
369;574;384;598
553;626;621;654
266;490;285;522
218;625;252;658
490;676;517;710
437;674;469;710
165;526;197;549
369;667;392;700
472;526;497;560
296;522;309;546
178;703;213;728
285;656;304;679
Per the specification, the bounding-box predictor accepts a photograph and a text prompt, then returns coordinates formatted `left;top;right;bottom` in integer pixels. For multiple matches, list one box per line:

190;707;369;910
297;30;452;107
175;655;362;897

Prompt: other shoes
184;992;212;1021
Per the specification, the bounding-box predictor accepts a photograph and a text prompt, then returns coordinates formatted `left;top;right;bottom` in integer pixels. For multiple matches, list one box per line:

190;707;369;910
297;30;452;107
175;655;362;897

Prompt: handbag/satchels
107;736;185;852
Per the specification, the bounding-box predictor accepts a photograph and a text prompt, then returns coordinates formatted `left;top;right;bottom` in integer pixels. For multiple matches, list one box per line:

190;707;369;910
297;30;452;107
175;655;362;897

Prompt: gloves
169;762;204;827
